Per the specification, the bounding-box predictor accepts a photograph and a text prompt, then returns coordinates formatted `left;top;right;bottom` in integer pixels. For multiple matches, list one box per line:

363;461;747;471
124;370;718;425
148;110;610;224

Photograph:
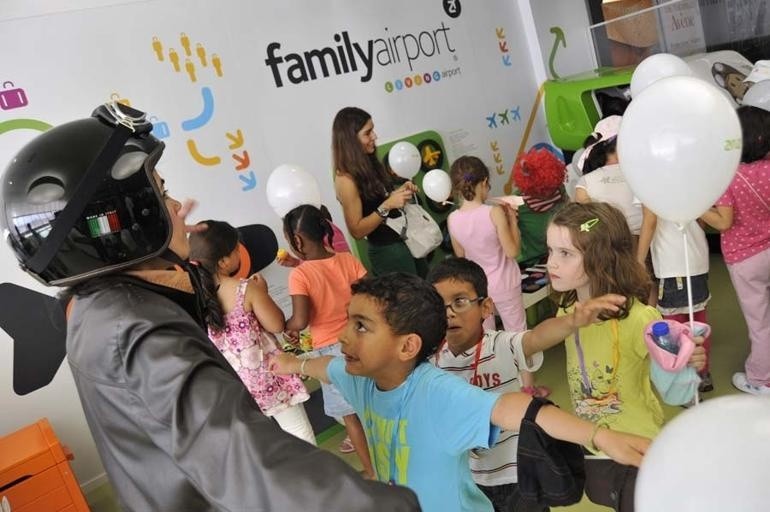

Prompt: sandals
520;385;549;404
339;434;355;453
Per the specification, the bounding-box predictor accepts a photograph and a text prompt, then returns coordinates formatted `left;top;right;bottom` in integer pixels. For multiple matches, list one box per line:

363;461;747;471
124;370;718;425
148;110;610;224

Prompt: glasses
443;296;486;315
24;102;153;278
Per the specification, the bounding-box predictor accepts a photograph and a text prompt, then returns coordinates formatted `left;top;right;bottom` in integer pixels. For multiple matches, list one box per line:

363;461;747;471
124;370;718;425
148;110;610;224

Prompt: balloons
422;168;453;203
388;140;423;182
630;53;695;101
633;393;770;512
619;79;742;229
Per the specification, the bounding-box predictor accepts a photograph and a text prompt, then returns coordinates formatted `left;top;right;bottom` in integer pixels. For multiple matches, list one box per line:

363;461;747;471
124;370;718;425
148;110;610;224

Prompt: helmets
1;117;178;289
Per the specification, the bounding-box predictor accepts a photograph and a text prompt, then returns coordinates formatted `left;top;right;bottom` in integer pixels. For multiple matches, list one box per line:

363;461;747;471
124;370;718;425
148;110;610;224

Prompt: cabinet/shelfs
0;413;95;511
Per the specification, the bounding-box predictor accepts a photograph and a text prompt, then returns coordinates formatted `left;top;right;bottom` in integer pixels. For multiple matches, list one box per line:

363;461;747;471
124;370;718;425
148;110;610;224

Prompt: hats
742;58;769;86
515;397;586;508
644;319;709;407
577;115;623;171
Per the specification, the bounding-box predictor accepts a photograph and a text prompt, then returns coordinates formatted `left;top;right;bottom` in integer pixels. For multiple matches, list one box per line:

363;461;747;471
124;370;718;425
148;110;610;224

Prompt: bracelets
300;357;313;376
587;419;611;452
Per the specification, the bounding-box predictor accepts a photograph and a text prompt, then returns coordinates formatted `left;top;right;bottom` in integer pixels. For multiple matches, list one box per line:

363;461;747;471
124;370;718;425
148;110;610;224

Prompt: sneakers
698;370;714;391
732;372;769;395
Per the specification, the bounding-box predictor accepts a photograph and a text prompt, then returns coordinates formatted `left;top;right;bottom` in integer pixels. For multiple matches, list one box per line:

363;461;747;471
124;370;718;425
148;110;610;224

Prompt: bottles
652;321;678;355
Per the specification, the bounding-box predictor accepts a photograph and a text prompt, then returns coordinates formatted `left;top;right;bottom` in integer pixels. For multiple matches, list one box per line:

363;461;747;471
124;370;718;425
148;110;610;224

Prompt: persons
283;202;380;482
446;155;553;400
515;116;712;393
425;257;628;512
330;105;423;275
188;218;318;449
696;104;770;394
274;215;352;269
267;269;654;511
543;201;708;457
0;99;422;510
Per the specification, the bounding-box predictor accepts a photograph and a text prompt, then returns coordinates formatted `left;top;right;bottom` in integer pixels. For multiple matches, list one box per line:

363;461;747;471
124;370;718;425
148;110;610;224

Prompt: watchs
374;206;390;219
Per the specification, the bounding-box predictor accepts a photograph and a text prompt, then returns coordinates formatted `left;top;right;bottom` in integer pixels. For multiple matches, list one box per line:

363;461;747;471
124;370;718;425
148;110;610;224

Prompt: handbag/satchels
385;190;443;262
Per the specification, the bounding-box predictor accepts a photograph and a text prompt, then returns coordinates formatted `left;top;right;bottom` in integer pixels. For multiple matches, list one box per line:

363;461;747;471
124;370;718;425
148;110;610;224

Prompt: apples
277;248;288;259
